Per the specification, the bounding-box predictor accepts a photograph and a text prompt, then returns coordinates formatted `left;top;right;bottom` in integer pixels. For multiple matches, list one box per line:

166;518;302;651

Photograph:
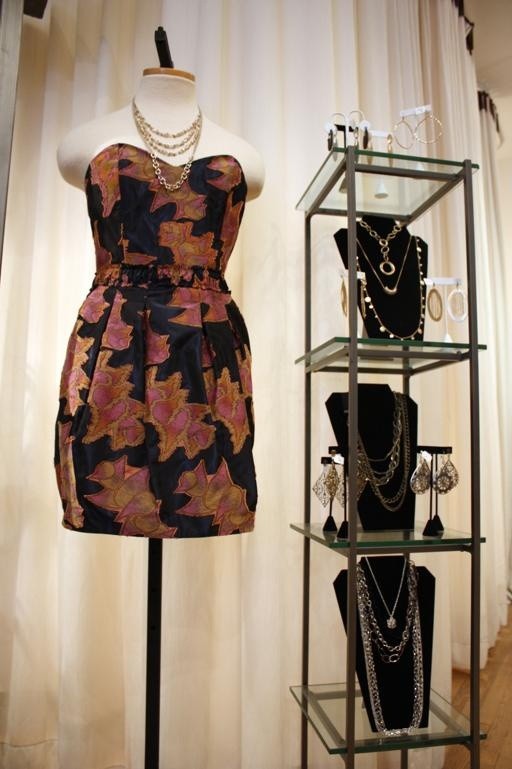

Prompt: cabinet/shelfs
286;145;496;768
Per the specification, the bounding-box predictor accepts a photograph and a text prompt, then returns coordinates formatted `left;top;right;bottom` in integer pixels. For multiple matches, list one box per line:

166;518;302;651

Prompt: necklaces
344;391;410;513
355;556;423;735
356;220;425;342
132;96;204;192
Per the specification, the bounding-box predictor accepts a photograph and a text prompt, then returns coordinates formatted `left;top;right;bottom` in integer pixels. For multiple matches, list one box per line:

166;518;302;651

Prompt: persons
56;68;265;537
